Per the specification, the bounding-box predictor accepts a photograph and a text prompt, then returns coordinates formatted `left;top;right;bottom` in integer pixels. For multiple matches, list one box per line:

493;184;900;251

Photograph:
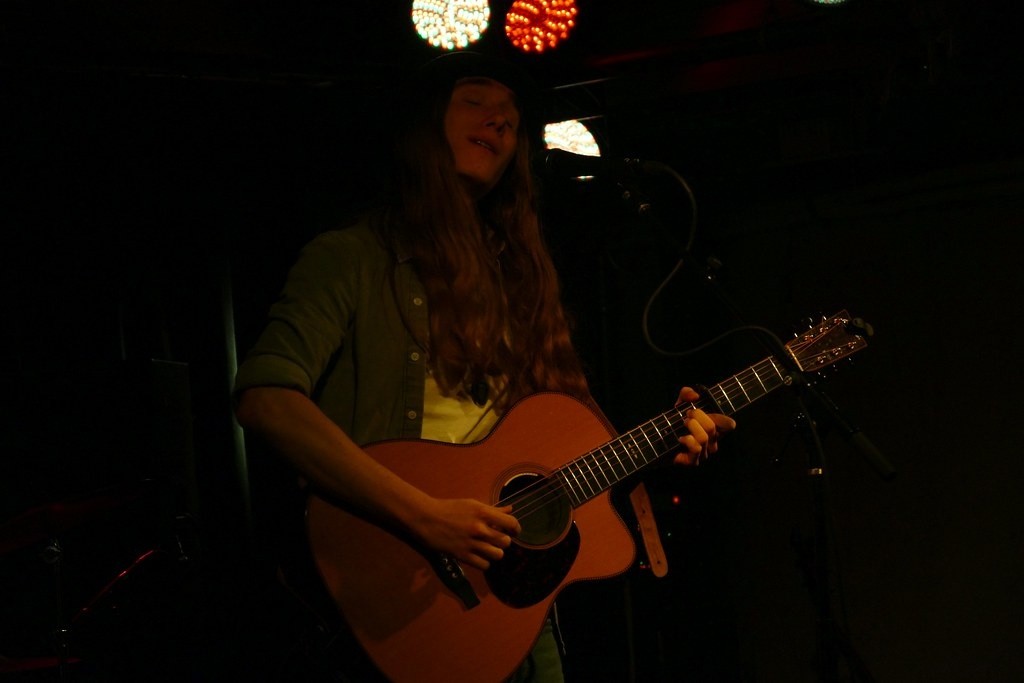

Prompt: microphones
533;149;642;181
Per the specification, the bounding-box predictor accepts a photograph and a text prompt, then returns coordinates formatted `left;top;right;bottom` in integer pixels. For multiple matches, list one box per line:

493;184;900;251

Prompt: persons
223;52;737;683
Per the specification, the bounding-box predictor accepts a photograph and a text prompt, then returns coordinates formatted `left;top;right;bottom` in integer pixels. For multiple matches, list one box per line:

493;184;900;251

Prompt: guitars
302;306;875;683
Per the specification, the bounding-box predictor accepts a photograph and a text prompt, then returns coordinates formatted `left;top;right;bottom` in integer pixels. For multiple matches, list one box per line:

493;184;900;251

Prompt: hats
398;50;551;148
